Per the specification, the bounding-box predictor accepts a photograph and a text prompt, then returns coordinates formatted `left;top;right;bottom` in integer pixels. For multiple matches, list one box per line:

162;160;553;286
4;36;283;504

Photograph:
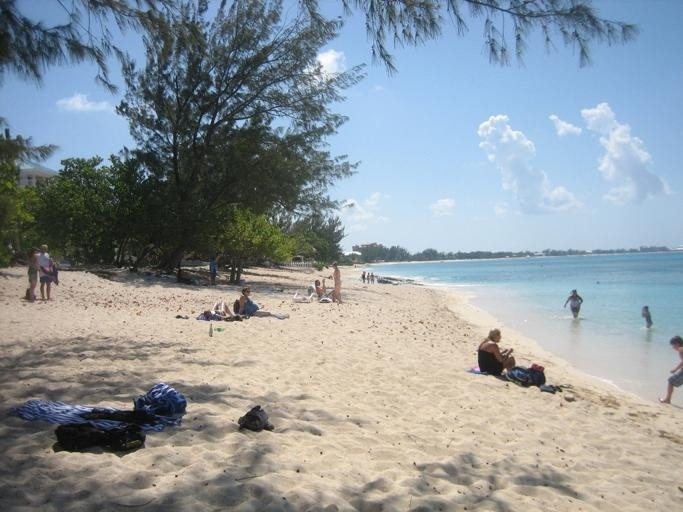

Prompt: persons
562;289;583;319
176;268;193;285
477;327;515;377
315;278;334;298
366;273;369;283
329;260;341;303
361;271;365;282
23;246;42;302
38;244;58;301
640;305;653;328
236;286;290;319
369;272;374;284
209;256;218;284
202;299;235;320
656;335;682;404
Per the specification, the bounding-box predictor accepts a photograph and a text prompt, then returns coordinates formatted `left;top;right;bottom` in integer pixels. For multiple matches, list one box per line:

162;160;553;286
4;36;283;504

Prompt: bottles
209;324;213;337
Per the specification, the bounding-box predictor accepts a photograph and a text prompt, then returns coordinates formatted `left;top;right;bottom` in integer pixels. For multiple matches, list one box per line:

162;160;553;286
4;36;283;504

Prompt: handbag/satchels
133;382;186;417
110;422;146;451
54;423;113;453
238;406;274;431
